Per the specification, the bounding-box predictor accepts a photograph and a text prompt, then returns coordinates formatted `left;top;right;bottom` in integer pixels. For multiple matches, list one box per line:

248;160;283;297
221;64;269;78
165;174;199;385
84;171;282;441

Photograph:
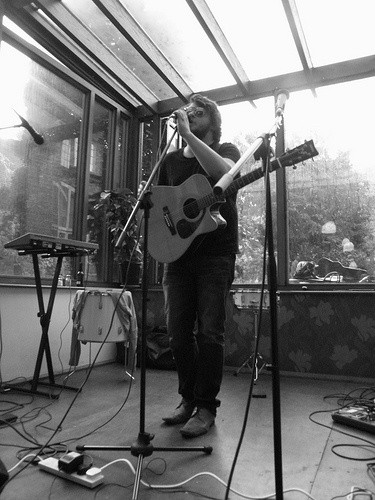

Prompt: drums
73;289;136;344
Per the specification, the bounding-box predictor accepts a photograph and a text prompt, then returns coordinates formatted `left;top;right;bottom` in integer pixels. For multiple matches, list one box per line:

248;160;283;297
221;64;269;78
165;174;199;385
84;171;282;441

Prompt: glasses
185;107;203;118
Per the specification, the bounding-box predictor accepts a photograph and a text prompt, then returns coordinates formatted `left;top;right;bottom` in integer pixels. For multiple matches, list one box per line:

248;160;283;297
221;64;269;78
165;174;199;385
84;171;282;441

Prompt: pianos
3;232;100;254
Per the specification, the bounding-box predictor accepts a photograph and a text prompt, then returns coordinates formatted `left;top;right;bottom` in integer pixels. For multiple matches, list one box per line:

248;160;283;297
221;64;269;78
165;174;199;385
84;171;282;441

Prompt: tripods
230;289;280;383
76;119;214;500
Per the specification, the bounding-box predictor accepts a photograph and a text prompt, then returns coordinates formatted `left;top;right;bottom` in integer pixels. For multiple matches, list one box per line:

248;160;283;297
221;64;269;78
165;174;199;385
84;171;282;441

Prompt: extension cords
38;457;104;489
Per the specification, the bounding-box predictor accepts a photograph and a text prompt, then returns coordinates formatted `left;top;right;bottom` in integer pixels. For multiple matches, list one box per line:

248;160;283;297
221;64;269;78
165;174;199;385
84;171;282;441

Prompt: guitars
134;138;320;265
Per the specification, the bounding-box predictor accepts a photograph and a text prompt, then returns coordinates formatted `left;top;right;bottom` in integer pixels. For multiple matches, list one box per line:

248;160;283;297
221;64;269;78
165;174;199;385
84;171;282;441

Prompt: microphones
275;89;290;115
17;114;44;145
171;114;178;118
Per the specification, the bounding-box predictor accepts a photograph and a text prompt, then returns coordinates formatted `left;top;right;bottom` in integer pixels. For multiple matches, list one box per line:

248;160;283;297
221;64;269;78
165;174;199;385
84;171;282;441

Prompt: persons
138;94;240;437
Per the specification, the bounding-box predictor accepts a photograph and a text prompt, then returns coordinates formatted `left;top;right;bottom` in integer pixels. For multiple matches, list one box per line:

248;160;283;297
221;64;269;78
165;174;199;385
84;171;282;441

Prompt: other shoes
162;399;195;423
180;407;215;436
